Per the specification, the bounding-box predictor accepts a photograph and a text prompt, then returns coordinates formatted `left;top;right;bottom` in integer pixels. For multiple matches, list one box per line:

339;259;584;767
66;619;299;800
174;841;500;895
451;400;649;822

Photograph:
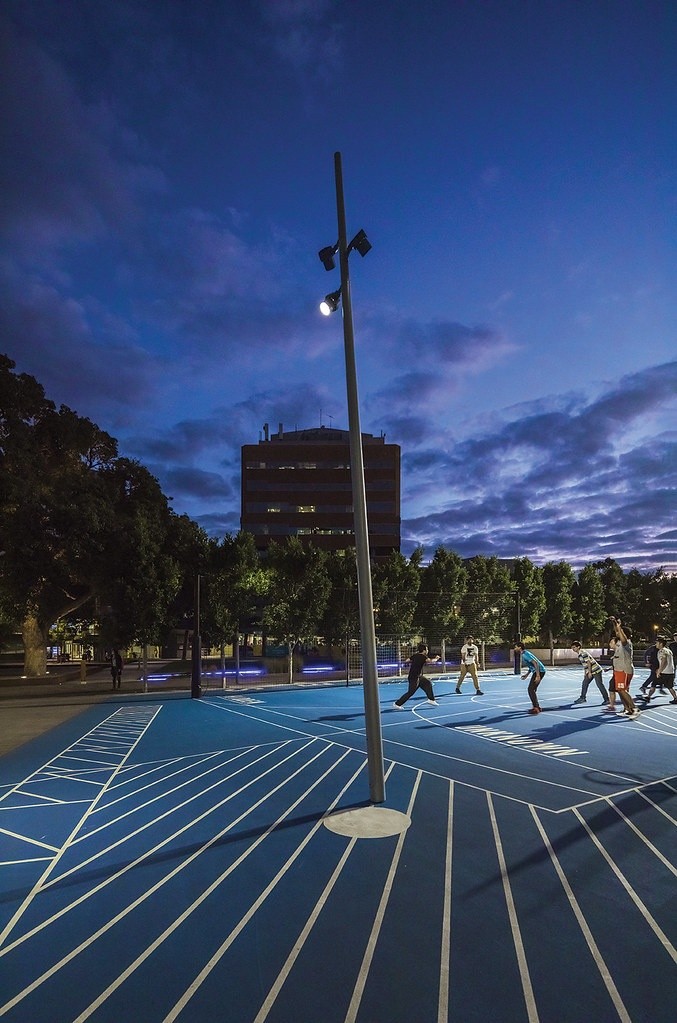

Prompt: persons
110;649;123;691
454;635;484;695
514;642;547;714
609;615;642;721
571;640;611;705
393;644;441;710
602;637;628;713
639;632;677;705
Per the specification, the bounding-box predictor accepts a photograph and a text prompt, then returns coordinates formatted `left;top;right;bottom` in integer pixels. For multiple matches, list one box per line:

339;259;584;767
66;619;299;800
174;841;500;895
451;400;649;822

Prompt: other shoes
574;698;586;704
639;697;650;702
528;706;542;714
476;690;483;695
392;702;404;710
454;688;462;694
602;700;610;705
639;687;648;695
659;689;667;694
669;699;677;704
428;699;440;706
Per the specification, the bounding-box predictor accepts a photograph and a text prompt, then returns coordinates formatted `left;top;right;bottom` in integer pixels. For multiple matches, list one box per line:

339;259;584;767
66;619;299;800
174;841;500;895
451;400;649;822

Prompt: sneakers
616;708;633;717
629;707;642;719
